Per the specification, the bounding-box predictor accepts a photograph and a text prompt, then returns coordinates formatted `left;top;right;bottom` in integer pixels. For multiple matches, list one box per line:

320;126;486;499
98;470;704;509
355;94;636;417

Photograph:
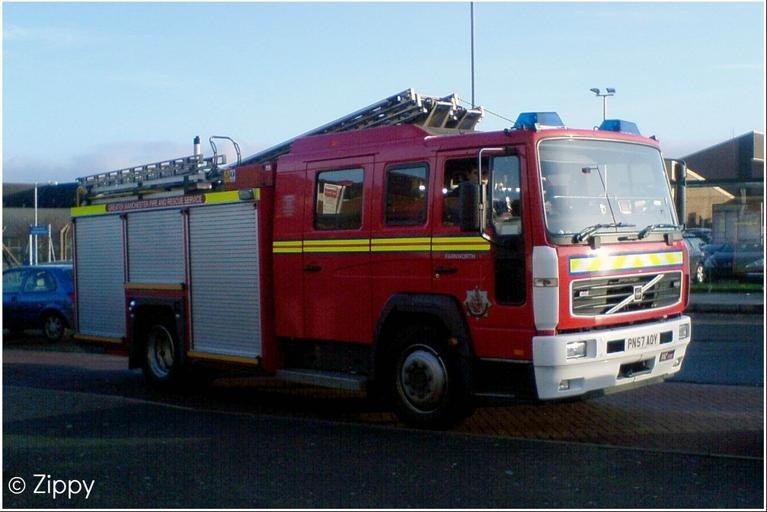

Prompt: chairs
29;273;53;293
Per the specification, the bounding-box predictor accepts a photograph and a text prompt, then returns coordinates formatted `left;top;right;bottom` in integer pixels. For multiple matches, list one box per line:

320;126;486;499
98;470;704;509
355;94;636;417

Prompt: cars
705;242;763;280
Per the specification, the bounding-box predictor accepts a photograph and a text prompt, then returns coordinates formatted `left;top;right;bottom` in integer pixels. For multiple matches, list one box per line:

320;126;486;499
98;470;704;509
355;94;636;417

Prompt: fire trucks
68;87;694;429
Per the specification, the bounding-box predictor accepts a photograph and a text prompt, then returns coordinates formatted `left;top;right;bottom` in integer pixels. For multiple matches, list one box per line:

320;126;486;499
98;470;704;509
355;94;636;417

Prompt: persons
443;159;511;226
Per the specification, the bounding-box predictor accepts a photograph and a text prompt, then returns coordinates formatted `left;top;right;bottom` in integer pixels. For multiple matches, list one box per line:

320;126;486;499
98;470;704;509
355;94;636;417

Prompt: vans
3;263;76;344
